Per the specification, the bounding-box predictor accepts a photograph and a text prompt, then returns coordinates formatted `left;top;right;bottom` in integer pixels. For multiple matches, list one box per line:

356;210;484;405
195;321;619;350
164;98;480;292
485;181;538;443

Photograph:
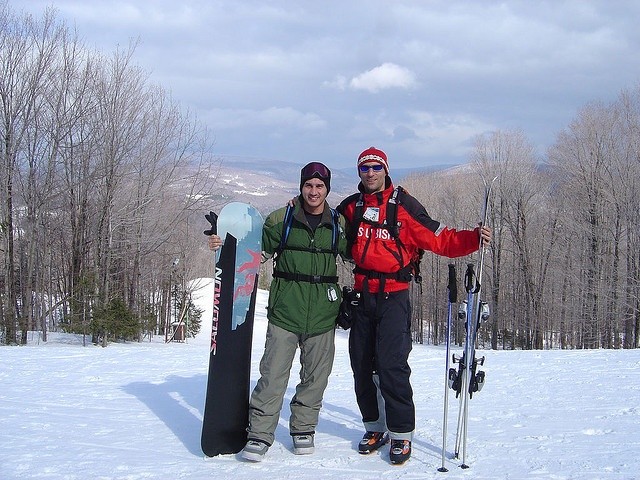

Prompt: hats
358;146;389;175
300;162;331;196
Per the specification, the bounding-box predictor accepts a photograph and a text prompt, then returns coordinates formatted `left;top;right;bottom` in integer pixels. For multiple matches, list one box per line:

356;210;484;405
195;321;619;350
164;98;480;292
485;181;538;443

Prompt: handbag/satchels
337;289;358;328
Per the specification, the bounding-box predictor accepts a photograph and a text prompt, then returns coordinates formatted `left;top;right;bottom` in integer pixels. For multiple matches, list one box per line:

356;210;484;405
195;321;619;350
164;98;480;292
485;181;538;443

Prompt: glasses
301;162;328;178
360;165;383;172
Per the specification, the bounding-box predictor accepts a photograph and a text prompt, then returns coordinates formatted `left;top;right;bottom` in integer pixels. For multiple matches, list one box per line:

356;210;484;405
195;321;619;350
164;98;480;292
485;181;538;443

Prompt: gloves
202;211;217;234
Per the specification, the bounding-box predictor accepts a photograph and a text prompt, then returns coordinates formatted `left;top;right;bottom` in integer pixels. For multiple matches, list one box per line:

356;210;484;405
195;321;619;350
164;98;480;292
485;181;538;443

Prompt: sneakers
242;441;268;462
358;431;390;454
292;435;314;454
390;440;412;464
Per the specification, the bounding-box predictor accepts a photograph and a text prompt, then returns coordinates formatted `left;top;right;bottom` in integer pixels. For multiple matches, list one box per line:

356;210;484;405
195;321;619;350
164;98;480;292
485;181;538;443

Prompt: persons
286;147;491;465
209;161;349;462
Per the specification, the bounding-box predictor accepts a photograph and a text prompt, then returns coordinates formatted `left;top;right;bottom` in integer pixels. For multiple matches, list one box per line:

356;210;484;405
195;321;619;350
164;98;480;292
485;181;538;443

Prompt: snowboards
200;202;264;458
